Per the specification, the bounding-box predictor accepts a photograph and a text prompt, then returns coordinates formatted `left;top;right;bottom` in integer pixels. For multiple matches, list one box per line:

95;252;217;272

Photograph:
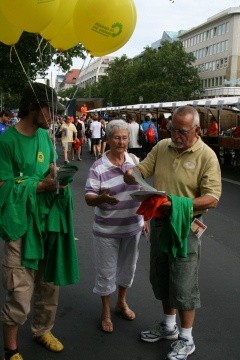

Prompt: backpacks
146;125;157;143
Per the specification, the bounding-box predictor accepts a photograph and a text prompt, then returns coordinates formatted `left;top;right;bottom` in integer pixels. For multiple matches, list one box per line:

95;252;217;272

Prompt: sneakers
3;353;23;360
39;331;64;351
140;319;180;342
166;334;196;360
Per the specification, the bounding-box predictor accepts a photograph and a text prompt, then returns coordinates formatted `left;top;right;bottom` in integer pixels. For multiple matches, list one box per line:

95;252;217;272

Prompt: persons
124;106;222;360
0;109;12;133
85;119;149;332
207;117;218;135
50;114;107;163
0;82;64;360
158;115;172;129
81;104;87;114
126;113;159;160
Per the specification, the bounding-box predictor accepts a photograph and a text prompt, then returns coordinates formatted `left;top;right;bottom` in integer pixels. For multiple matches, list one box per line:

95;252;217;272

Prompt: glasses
170;125;197;135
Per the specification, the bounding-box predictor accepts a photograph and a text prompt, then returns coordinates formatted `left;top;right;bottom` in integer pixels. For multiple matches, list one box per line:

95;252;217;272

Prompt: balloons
0;0;137;58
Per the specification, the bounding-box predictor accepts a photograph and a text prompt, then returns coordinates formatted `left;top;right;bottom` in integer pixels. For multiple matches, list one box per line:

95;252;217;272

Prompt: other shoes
114;302;135;321
101;314;113;334
64;160;69;165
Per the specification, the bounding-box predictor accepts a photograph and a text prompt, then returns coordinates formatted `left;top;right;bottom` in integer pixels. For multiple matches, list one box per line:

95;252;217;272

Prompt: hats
20;82;66;111
